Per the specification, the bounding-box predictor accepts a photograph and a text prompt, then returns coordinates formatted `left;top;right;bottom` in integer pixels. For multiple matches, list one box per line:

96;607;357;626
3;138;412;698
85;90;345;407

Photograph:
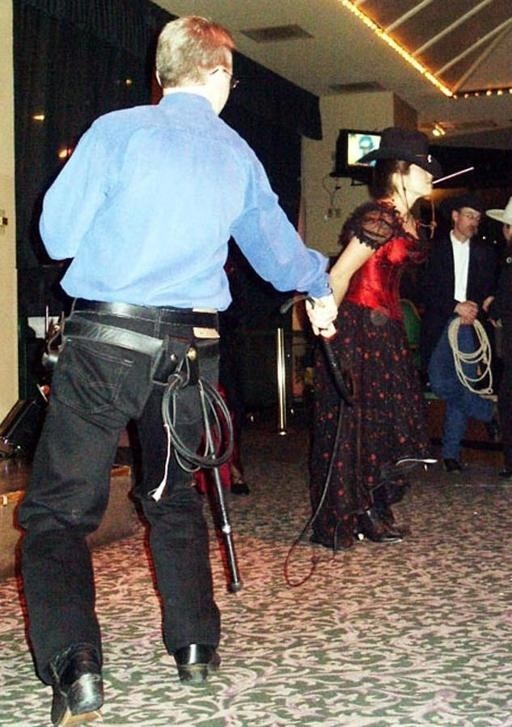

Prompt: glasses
458;212;481;221
213;68;240;89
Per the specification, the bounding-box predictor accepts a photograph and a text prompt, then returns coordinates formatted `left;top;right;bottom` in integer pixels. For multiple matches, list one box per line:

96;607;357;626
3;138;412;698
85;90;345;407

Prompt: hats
486;196;512;226
439;194;488;223
355;126;443;179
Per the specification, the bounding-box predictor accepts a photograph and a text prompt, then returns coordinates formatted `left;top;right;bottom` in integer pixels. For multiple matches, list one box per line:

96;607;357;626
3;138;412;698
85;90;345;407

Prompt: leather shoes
51;644;104;727
499;464;512;478
373;504;412;535
358;506;404;544
174;642;221;685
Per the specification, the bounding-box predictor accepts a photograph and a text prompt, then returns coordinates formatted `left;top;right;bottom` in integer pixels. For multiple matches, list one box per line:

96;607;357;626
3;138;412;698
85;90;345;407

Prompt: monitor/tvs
335;129;382;170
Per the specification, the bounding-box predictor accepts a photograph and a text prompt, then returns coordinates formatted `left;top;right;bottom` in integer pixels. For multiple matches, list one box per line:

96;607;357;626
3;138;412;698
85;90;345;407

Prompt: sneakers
444;458;462;474
485;404;504;444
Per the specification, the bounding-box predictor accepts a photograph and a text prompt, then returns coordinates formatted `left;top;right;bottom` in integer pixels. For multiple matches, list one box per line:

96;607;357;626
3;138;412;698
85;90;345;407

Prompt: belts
74;298;218;329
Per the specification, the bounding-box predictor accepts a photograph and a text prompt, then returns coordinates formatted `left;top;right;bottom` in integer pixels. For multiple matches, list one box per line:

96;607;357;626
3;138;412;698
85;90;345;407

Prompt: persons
191;238;251;496
418;192;502;474
306;126;435;551
18;14;339;727
485;194;512;477
